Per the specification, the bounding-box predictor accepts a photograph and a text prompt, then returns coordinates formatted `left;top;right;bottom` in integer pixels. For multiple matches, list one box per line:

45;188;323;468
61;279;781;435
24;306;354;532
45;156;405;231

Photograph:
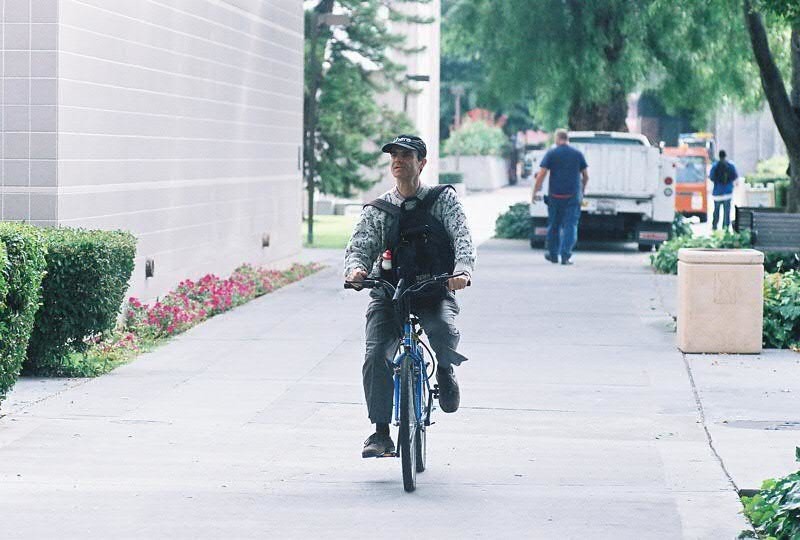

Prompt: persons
345;134;475;457
531;128;589;266
709;150;738;232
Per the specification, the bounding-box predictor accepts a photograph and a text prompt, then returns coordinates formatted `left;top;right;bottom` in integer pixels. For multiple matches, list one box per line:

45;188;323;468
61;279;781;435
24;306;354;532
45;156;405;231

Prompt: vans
520;141;546;179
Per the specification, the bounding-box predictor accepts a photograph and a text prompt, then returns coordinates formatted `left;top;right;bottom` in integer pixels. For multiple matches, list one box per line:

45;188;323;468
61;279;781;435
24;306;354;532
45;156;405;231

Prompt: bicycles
344;270;471;493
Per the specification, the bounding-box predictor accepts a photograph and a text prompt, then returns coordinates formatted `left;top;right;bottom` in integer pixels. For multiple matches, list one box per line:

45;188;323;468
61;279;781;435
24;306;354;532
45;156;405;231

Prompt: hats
382;134;427;157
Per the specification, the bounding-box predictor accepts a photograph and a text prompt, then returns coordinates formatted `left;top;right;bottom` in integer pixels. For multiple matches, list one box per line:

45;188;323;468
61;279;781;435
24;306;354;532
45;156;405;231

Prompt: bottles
380;249;392;270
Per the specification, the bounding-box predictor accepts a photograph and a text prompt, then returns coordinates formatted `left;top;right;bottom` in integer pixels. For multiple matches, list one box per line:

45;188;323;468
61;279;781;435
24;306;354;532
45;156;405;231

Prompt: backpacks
363;184;457;306
714;160;731;184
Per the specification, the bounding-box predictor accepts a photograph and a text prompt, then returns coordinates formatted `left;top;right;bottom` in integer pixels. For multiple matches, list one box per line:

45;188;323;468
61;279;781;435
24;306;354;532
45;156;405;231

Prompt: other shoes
562;258;573;264
546;253;558;262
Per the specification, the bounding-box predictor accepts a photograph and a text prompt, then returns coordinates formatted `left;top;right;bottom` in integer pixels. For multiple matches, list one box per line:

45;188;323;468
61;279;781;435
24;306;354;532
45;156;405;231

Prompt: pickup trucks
531;130;676;251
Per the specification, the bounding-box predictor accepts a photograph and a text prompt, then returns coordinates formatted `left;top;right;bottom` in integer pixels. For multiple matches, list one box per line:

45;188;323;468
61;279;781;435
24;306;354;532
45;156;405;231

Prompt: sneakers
361;432;395;458
435;366;459;413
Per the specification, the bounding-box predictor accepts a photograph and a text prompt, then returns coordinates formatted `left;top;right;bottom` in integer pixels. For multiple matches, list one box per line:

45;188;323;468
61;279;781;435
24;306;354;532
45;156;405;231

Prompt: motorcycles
664;146;708;223
